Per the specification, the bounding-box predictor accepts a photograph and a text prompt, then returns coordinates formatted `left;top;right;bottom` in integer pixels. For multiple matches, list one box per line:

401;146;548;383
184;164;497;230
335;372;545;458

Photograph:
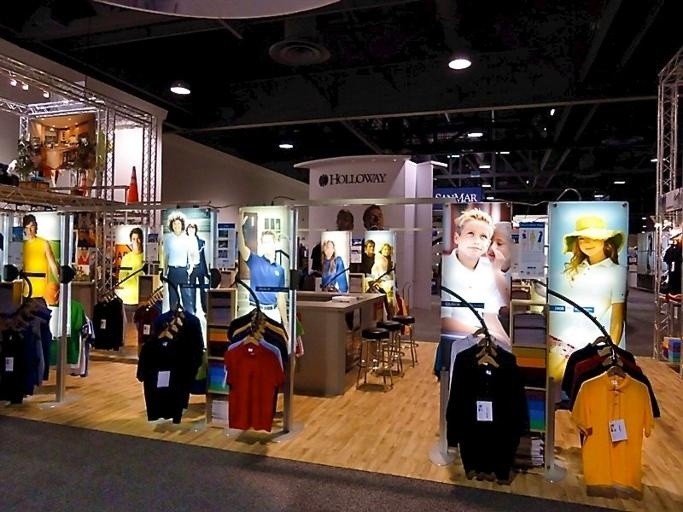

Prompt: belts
250;301;277;310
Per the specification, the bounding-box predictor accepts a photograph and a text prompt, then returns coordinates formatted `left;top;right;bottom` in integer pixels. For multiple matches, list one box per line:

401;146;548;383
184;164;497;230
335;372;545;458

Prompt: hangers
244;309;265;346
0;296;39;331
591;327;625;378
158;309;185;340
145;287;163;307
101;288;116;300
473;318;498;368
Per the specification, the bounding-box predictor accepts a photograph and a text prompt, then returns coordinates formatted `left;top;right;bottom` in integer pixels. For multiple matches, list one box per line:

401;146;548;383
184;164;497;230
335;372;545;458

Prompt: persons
486;221;512;307
322;240;348;293
336;208;355;231
78;245;91;265
548;215;627;359
363;204;384;231
363;239;393;280
441;208;512;347
235;207;288;332
118;211;209;319
22;214;61;306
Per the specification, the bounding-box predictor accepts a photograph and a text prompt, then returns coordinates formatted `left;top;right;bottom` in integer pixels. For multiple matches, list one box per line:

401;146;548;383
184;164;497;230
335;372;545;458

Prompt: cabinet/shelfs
511;299;549;476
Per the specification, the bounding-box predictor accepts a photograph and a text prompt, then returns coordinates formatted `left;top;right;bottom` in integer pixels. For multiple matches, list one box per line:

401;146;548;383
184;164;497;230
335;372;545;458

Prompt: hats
560;216;627;256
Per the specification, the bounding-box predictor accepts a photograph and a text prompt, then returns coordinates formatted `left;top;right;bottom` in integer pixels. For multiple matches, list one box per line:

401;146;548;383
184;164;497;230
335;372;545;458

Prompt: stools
356;315;418;391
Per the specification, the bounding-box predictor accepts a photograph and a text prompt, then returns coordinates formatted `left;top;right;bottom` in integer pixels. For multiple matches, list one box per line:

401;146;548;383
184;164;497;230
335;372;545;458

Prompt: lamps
169;80;191;97
